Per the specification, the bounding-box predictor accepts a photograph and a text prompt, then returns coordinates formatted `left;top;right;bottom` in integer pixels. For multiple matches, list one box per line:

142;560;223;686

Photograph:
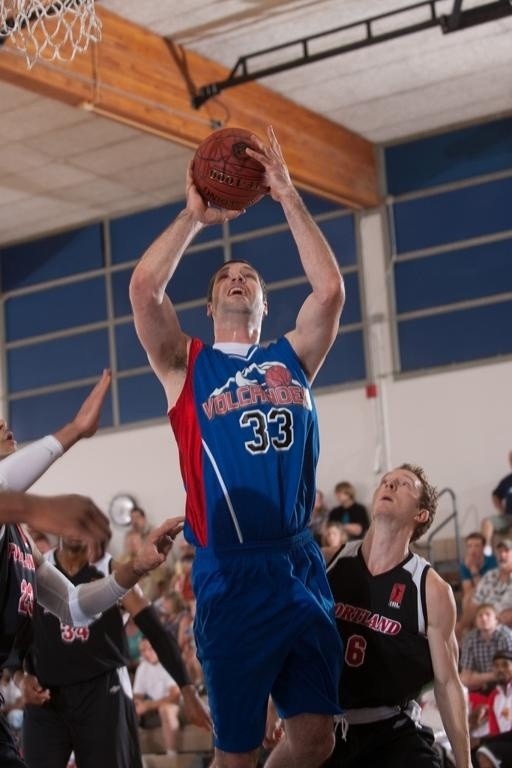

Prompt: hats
495;538;512;551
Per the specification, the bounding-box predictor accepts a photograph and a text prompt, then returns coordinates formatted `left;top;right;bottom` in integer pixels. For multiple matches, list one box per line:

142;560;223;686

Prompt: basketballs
191;127;268;210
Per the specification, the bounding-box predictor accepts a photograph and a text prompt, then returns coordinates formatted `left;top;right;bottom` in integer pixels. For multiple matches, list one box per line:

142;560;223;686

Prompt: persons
263;464;473;767
309;481;369;561
0;368;211;765
419;452;512;767
128;125;346;767
